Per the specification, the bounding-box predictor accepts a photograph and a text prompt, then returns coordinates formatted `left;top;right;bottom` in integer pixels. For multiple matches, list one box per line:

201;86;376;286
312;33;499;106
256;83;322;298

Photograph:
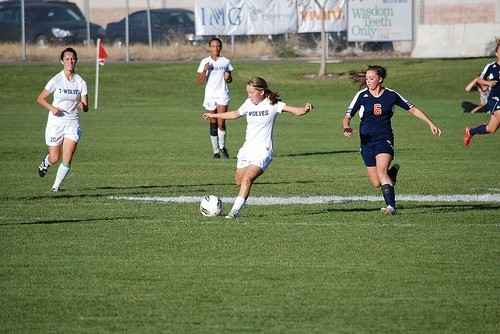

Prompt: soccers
199;195;224;217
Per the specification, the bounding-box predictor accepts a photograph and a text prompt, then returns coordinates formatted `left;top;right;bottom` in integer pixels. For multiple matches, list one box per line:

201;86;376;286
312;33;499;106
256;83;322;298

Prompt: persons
461;74;491;114
37;47;89;192
197;38;234;158
201;76;314;217
463;39;500;146
343;65;442;215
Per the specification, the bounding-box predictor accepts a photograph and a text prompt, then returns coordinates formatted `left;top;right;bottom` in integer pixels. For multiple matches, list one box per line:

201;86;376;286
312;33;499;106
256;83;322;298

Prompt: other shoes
38;154;52;177
224;210;238;219
380;205;396;216
213;154;220;159
389;163;400;186
51;188;60;192
221;147;230;158
463;126;473;146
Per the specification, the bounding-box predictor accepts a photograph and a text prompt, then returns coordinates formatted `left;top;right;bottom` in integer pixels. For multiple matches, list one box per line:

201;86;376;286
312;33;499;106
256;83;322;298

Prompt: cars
106;8;224;48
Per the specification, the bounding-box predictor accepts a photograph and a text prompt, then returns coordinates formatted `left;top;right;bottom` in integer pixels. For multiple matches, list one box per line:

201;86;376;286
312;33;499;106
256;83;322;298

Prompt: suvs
0;0;104;47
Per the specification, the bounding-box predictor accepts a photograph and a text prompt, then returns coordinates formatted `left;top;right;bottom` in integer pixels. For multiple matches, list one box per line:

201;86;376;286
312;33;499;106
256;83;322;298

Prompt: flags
100;42;108;66
